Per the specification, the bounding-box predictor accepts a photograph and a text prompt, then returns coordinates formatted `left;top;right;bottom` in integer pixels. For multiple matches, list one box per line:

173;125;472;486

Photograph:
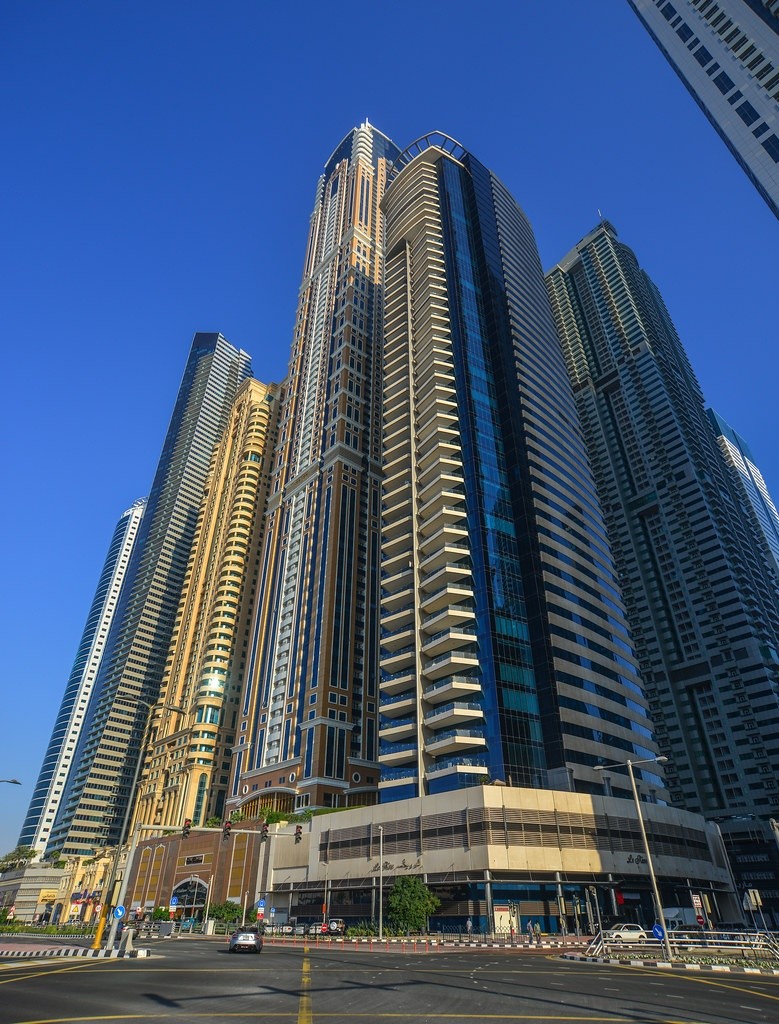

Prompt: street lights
378;826;384;941
592;756;678;959
92;694;185;948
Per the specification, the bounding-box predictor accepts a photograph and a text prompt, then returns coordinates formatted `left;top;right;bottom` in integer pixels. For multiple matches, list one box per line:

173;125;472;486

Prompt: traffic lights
295;825;302;845
222;821;233;843
261;822;269;844
181;818;192;841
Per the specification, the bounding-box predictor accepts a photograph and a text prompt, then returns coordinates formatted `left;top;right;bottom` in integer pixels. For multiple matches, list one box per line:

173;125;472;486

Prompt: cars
293;923;310;934
229;926;268;952
308;922;324;934
280;923;294;934
264;923;278;934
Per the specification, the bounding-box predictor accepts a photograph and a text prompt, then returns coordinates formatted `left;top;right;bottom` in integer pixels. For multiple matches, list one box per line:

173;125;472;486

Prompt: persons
465;918;472;934
585;922;599;936
527;919;533;944
623;927;628;931
533;920;542;944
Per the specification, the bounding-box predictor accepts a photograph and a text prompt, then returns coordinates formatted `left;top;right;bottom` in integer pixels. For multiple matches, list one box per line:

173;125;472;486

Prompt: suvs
329;919;347;935
601;923;648;944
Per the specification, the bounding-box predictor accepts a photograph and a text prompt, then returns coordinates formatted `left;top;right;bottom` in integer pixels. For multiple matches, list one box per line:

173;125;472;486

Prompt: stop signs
322;924;329;932
697;915;705;925
